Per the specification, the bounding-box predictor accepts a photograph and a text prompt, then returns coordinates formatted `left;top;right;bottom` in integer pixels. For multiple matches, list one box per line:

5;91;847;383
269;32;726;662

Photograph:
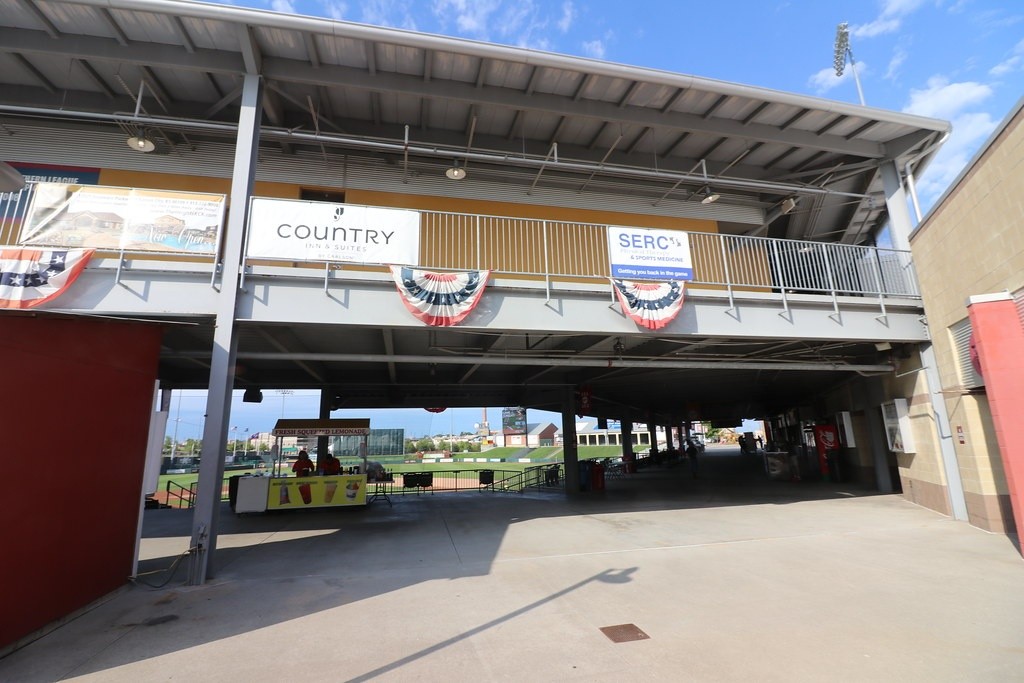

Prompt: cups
299;484;312;504
324;484;337;503
346;487;359;501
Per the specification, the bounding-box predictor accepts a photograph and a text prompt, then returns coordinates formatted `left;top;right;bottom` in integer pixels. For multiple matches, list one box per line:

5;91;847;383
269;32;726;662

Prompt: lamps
702;183;719;204
243;388;264;403
445;157;467;180
127;128;156;153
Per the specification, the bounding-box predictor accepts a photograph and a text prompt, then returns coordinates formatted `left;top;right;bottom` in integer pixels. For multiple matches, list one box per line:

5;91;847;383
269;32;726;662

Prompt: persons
739;436;745;453
757;436;763;448
292;451;314;476
320;454;340;475
686;444;698;480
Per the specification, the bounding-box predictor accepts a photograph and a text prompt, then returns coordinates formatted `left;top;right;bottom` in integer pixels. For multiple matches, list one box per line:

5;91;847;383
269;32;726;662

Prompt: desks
366;479;395;508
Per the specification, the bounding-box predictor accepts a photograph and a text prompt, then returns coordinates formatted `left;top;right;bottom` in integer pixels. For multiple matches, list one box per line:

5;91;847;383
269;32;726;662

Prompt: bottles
340;461;353;475
383;468;393;481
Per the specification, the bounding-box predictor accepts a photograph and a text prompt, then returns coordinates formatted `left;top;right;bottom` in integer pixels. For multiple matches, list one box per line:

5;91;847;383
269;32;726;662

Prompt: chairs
479;470;495;493
403;472;434;497
